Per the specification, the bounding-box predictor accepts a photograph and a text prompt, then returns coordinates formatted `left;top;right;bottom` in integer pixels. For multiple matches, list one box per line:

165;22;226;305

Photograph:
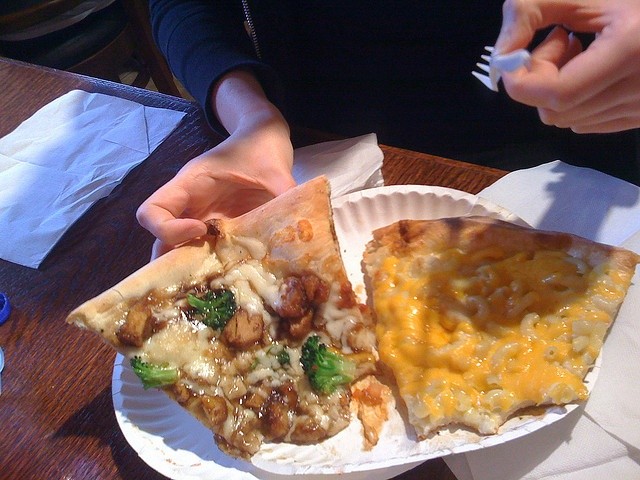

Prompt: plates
246;185;603;475
111;185;423;478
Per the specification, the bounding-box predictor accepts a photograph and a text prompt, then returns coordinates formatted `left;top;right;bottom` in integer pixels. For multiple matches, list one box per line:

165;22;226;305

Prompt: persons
135;1;639;263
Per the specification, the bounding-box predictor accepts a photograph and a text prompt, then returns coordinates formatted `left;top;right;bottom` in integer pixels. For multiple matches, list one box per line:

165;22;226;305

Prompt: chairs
1;1;184;103
0;56;637;480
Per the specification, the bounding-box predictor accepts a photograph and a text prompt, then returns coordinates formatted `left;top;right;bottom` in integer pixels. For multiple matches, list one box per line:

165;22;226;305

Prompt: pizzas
65;175;387;460
363;214;640;441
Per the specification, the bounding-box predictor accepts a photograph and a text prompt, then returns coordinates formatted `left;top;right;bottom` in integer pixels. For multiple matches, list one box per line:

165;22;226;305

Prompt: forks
472;45;530;92
